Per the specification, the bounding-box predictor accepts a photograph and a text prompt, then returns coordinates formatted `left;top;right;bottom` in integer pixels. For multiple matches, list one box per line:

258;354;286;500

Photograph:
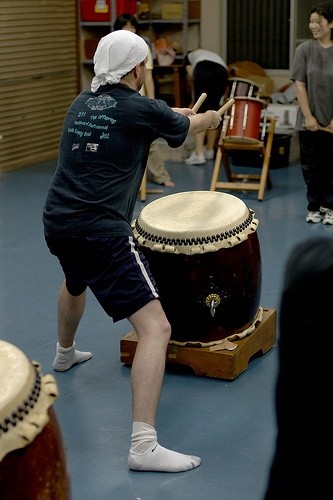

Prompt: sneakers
305;211;323;224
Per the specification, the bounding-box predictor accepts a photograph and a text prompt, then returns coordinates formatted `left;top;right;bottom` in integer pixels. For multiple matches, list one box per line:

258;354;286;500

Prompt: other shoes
184;150;206;165
203;147;214;160
322;209;333;225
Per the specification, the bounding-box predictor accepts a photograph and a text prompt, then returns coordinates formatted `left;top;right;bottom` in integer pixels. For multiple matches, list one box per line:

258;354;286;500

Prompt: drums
222;95;269;145
0;339;72;500
130;189;264;347
219;77;263;107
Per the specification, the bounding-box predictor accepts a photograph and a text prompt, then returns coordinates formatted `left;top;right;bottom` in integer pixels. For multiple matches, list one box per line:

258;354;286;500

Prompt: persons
43;29;221;472
114;12;174;187
185;48;228;164
263;237;333;500
291;3;333;224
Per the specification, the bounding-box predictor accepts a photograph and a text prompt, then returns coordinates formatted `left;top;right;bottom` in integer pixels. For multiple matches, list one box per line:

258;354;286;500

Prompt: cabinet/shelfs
76;0;200;108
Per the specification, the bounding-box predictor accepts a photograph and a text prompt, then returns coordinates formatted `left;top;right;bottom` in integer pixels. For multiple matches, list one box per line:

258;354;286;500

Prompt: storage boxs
259;103;303;136
80;0;138;22
231;134;292;169
160;4;184;19
